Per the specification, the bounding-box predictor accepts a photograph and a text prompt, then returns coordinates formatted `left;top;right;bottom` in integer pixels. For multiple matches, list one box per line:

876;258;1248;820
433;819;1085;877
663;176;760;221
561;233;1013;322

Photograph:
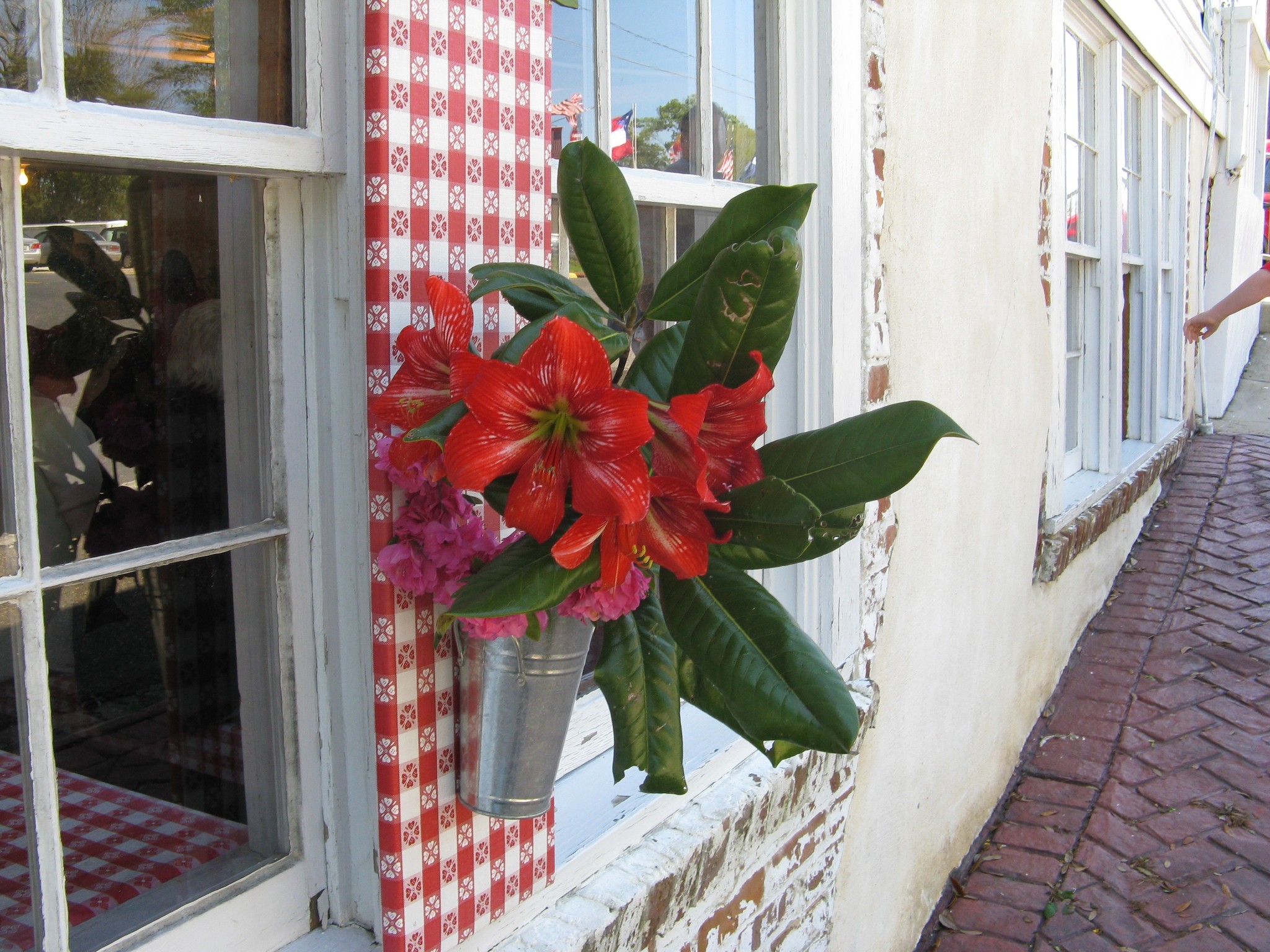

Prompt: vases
455;612;593;818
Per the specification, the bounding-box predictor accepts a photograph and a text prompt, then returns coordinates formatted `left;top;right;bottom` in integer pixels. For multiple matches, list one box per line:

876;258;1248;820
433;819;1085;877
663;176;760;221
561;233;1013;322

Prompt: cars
100;225;136;269
33;229;122;272
23;232;42;272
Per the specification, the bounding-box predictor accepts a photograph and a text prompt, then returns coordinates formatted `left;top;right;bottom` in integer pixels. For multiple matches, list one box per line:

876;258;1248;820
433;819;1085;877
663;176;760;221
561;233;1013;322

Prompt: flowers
379;141;980;794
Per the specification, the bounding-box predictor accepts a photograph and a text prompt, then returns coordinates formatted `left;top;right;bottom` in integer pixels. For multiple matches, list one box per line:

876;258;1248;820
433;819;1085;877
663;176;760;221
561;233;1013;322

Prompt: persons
1182;200;1270;344
0;338;106;744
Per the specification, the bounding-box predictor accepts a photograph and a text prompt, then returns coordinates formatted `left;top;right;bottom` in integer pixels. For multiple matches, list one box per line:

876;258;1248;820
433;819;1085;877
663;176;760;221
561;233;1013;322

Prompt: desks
0;749;249;952
